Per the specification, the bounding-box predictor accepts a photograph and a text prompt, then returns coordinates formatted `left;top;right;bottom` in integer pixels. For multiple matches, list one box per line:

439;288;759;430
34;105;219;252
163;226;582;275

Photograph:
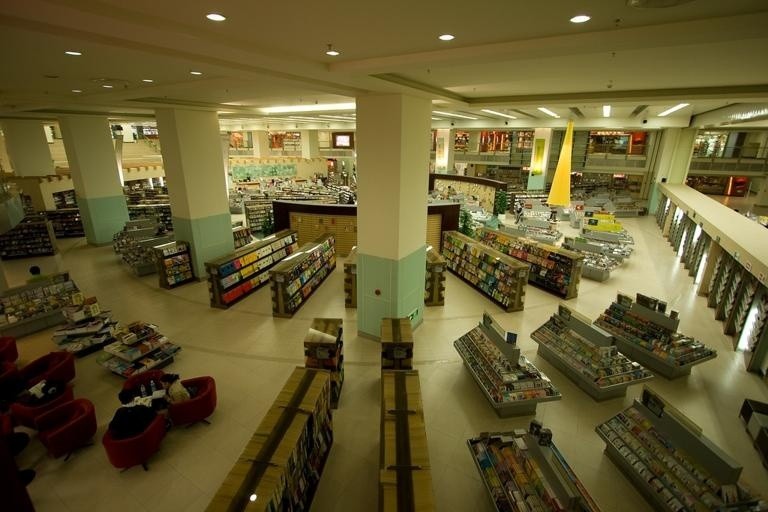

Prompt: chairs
1;351;218;473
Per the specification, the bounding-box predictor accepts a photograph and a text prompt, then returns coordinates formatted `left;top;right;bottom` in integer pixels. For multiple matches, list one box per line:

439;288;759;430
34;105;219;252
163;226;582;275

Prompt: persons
158;373;191;433
25;377;65;405
107;385;157;442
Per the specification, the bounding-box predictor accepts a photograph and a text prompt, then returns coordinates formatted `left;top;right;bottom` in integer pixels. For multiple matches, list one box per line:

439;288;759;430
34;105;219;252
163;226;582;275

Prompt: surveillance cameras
505;121;508;125
643;120;647;123
451;122;454;125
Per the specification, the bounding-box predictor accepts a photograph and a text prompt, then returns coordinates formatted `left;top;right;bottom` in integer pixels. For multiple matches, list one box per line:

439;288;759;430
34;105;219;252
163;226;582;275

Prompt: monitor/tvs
336;135;350;147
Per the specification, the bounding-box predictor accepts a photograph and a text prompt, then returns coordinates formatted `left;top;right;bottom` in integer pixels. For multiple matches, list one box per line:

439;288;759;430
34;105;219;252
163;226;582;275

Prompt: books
134;396;152;408
265;325;348;512
49;325;182;381
152;388;166;400
28;381;47;399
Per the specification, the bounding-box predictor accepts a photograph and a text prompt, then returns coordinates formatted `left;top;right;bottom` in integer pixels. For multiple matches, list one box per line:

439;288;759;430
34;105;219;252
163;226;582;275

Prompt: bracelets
166;397;171;403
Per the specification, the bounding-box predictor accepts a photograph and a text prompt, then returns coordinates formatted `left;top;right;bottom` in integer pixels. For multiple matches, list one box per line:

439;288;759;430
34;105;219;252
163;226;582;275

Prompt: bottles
140;380;156;398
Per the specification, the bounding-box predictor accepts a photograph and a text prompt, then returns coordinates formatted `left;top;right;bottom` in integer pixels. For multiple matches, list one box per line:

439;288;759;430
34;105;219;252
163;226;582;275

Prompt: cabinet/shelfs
656;195;766;376
204;226;336;319
453;292;762;510
229;180;356;234
113;178;196;289
206;317;345;510
1;187;86;260
377;317;425;510
343;248;358;310
427;166;635;312
3;273;181;379
